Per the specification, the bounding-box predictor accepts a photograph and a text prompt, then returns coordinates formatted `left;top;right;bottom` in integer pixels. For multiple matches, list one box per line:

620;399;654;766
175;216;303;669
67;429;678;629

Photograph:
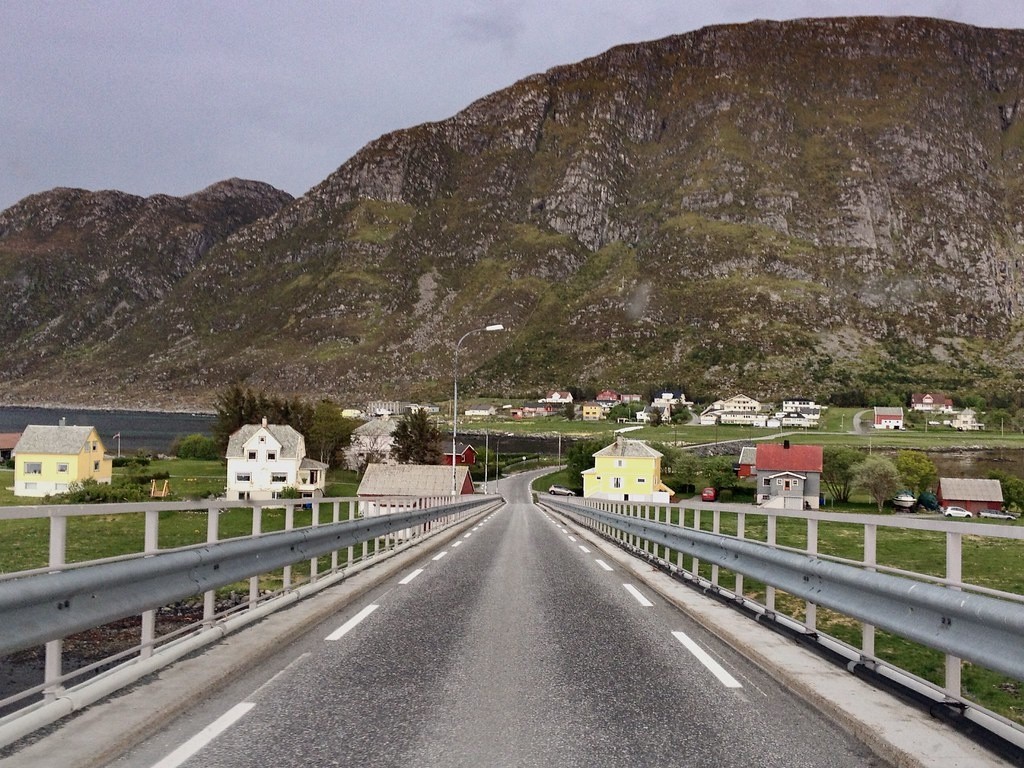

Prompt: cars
977;509;1017;520
944;506;973;518
549;485;575;497
702;488;718;502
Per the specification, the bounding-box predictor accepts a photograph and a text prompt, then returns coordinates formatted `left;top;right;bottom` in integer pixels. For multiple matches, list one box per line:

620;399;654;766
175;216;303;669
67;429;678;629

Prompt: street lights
496;433;515;494
451;324;504;500
552;430;561;472
484;404;512;495
